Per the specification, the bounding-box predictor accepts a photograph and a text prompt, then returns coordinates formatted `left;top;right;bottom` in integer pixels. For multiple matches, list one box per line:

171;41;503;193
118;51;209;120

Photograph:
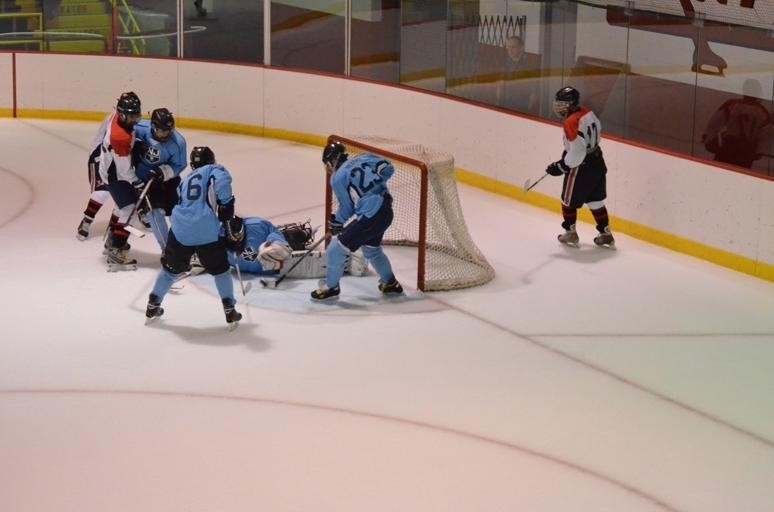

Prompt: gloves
132;180;149;198
147;166;166;182
545;161;569;178
216;197;235;220
327;214;344;234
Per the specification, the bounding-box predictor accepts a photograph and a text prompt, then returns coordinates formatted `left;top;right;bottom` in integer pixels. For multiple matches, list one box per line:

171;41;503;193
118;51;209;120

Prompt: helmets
151;108;174;129
117;91;141;114
190;146;215;165
221;215;243;234
554;86;581;115
323;140;346;168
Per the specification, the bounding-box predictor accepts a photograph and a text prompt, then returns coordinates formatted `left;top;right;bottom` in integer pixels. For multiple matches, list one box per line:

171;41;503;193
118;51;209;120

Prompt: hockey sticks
233;252;252;296
167;265;206;290
524;173;548;191
122;176;155;238
260;231;332;288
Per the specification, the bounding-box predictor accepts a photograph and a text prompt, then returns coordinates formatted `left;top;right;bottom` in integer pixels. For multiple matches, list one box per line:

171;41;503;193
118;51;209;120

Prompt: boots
146;294;164;317
310;282;341;299
104;237;131;250
137;209;151;227
106;249;137;265
222;297;242;323
558;231;579;245
377;276;404;293
76;214;93;237
593;234;617;246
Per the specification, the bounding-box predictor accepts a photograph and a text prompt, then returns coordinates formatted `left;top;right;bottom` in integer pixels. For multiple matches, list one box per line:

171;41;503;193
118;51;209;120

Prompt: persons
146;146;243;323
546;87;614;244
702;78;774;169
132;109;187;223
311;142;403;299
78;92;145;264
185;217;313;274
496;36;539;114
